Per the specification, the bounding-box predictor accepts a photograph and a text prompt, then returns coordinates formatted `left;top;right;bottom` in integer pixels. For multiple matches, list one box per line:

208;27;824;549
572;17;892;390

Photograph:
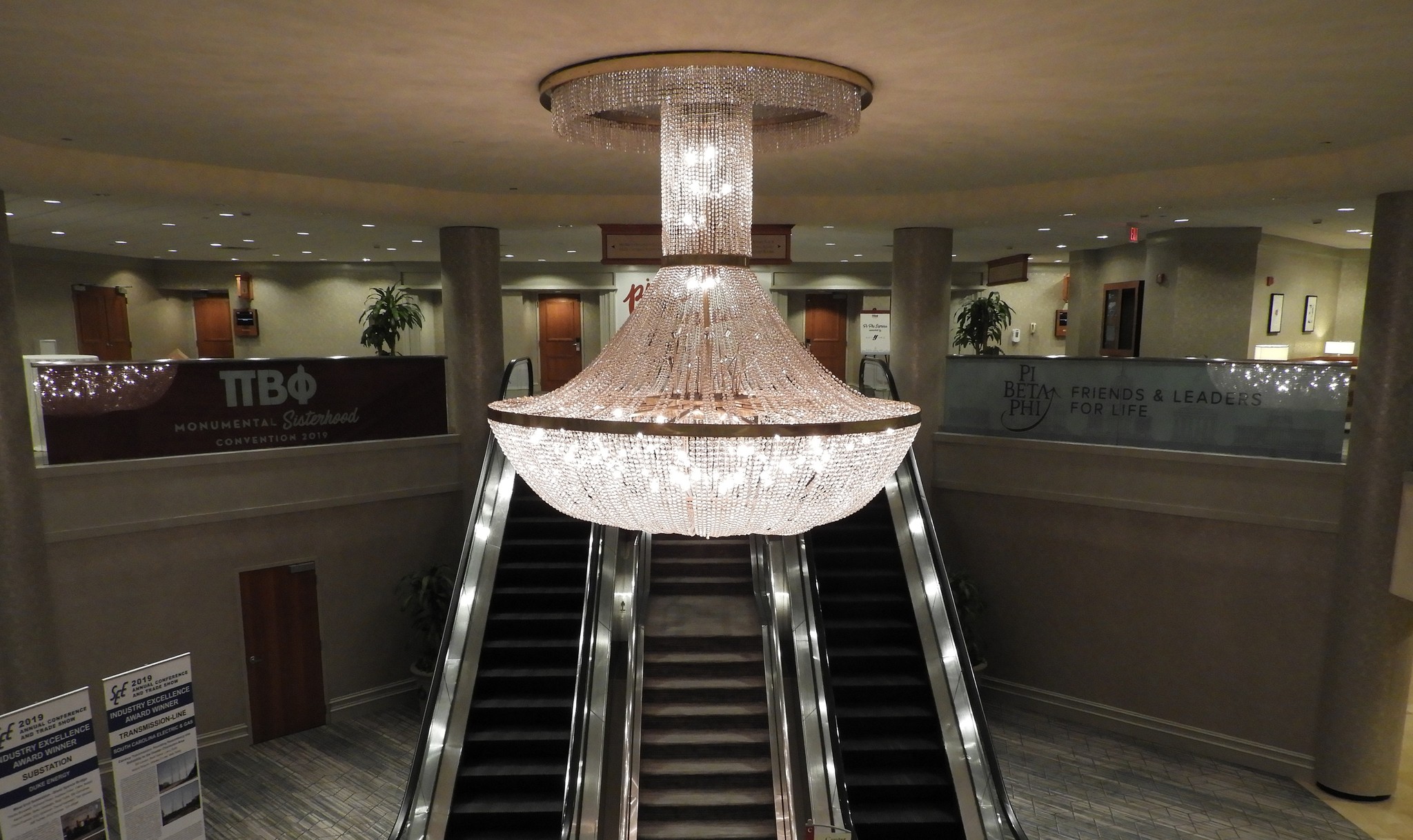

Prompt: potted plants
393;564;455;710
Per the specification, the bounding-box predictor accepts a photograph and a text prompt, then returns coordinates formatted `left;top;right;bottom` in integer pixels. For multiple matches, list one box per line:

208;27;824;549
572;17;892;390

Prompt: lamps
486;53;923;537
1325;341;1355;357
1255;345;1289;361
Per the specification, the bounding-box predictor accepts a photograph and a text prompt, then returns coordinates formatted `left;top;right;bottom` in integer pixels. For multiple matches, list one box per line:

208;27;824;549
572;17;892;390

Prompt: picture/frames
1302;295;1318;332
1267;293;1284;333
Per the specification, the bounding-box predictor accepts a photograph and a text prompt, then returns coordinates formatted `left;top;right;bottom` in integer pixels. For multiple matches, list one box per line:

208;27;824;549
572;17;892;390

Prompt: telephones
1030;323;1037;334
